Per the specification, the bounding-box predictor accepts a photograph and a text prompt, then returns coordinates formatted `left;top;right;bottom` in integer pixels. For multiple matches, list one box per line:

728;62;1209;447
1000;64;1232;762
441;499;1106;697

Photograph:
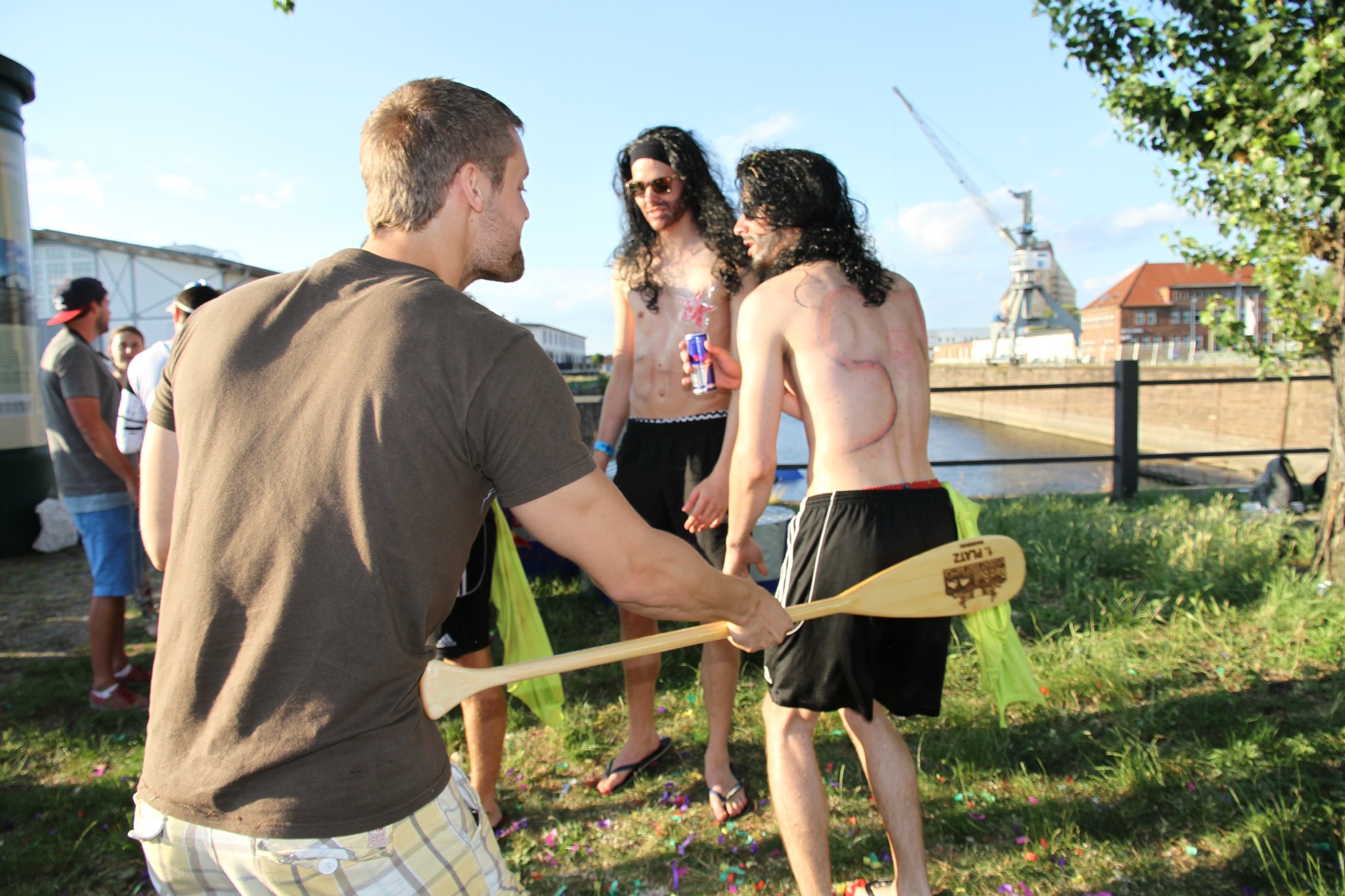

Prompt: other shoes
471;799;511;837
89;664;152;710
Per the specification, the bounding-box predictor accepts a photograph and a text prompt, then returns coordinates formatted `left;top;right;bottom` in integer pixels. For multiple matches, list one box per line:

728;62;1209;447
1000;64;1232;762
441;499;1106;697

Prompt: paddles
420;534;1026;720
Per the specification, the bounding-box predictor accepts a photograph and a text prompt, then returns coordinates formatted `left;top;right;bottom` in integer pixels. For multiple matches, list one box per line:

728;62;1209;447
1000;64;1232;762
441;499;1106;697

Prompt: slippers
594;736;673;796
708;762;754;824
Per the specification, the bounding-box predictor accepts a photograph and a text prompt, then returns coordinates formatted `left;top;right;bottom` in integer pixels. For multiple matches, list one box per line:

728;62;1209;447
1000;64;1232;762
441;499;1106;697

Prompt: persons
680;148;959;896
39;277;225;711
589;127;767;823
433;506;513;833
127;75;798;896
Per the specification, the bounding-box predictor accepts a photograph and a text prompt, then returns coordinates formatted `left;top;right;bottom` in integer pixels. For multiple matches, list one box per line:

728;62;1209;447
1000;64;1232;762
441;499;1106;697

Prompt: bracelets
593;440;615;456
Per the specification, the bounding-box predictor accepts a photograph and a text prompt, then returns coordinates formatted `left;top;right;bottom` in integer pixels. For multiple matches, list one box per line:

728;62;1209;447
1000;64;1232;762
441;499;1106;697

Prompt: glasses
625;174;677;198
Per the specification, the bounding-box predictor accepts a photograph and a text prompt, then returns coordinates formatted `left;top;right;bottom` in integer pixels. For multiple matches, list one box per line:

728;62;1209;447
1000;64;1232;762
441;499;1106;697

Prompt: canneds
685;332;718;395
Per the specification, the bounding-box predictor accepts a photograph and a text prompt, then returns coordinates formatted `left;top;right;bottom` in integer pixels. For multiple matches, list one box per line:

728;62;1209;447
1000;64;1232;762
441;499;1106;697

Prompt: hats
165;282;205;314
46;277;108;326
